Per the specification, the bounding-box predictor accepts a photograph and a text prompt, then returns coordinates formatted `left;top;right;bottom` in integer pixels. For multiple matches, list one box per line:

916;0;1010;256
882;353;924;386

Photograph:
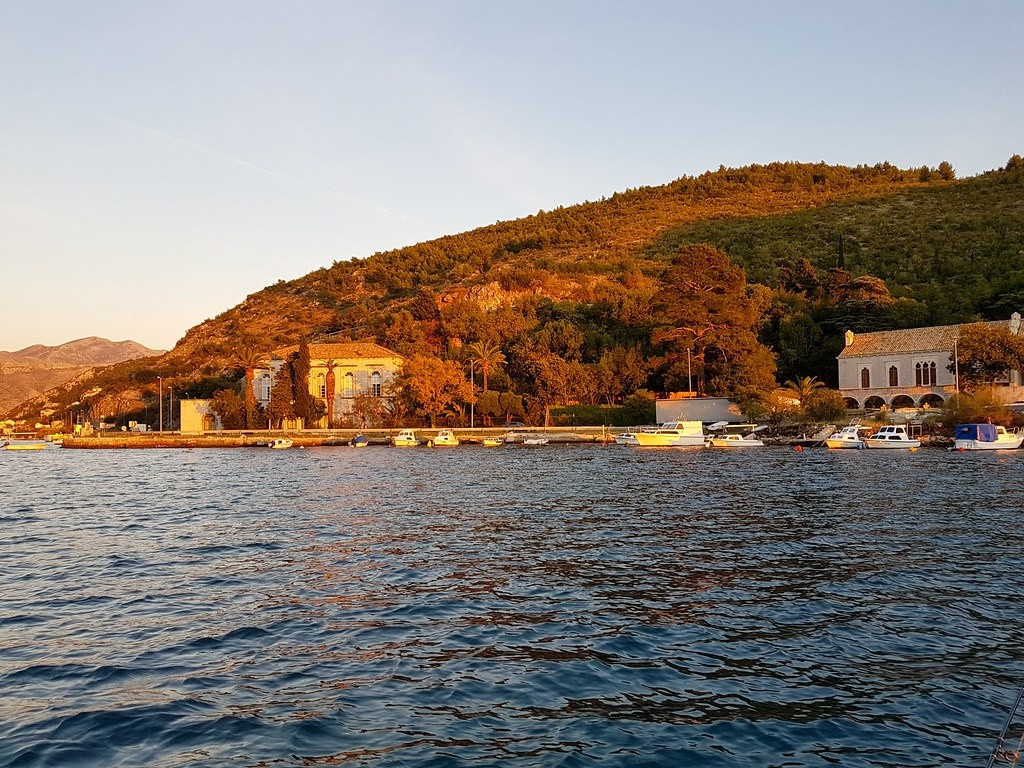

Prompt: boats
391;431;422;447
4;432;48;450
348;408;370;448
613;432;640;446
522;436;550;448
634;421;706;447
704;424;765;448
864;424;922;449
947;421;1024;457
483;438;505;449
433;431;460;448
271;438;294;449
825;419;873;449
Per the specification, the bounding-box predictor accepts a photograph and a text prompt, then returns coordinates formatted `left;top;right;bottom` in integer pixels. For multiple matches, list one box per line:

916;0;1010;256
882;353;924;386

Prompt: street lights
169;385;174;430
953;335;962;410
144;406;148;425
52;409;85;429
469;358;475;428
267;363;273;435
157;376;163;436
686;347;692;399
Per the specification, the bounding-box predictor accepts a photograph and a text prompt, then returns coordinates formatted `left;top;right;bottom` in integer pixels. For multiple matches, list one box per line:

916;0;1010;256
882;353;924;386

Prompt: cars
500;422;525;428
